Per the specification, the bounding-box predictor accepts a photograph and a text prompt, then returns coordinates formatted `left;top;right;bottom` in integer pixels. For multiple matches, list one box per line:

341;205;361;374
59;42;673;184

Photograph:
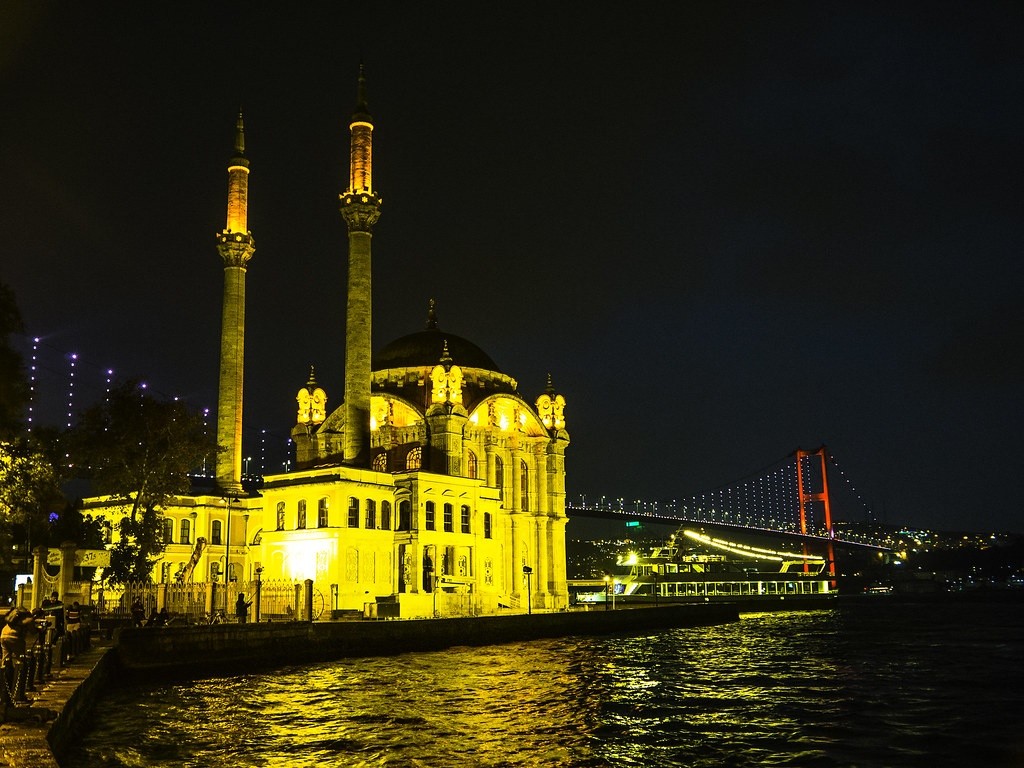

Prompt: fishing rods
232;577;266;620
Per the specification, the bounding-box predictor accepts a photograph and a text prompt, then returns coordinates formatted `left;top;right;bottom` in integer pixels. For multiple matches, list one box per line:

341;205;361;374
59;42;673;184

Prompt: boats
575;522;838;603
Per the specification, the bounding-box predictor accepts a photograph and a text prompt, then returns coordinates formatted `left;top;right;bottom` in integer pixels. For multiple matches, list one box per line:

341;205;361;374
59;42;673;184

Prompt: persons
0;605;45;667
42;591;64;611
144;607;170;627
66;601;87;624
236;593;253;623
130;596;146;628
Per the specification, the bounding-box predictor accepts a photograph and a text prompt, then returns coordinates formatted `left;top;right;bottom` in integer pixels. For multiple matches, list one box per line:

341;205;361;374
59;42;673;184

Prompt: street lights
283;460;291;474
617;498;623;511
244;457;251;474
219;490;240;611
650;502;657;515
634;500;641;515
603;575;609;611
580;494;586;502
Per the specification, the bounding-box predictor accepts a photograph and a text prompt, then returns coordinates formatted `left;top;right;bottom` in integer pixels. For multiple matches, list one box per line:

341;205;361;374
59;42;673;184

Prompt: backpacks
4;607;33;626
